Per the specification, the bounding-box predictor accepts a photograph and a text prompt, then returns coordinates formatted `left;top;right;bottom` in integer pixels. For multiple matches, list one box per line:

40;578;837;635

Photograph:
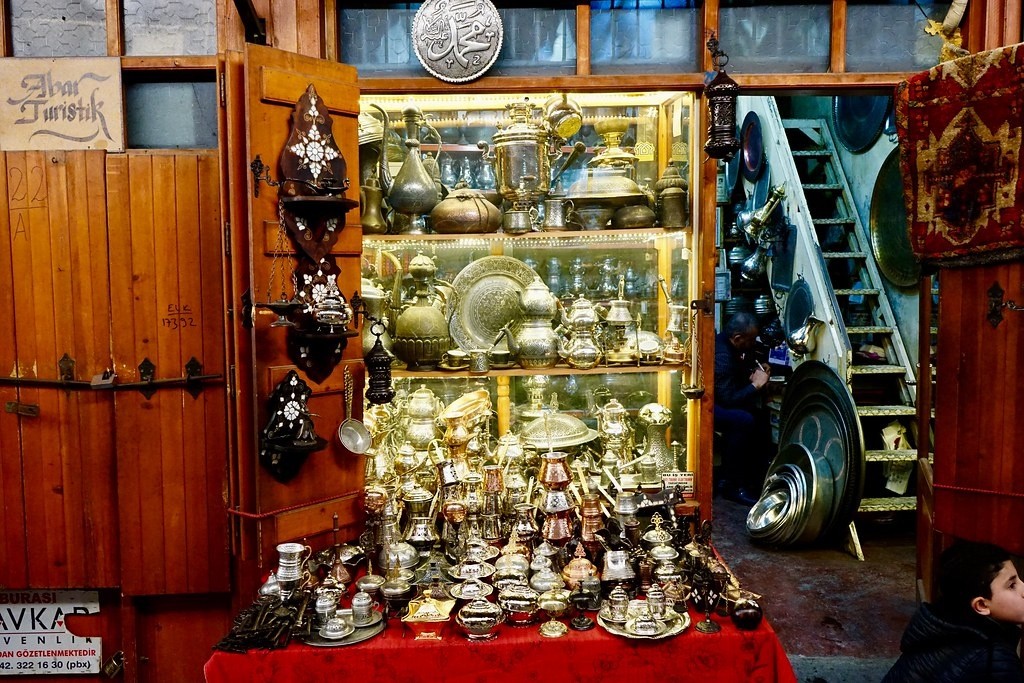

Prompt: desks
204;562;798;682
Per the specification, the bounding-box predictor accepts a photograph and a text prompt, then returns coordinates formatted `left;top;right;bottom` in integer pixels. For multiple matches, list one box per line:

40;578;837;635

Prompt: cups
645;584;666;618
441;350;466;366
504;211;531;234
543;198;574;231
351;591;380;624
640;453;657;480
469;350;490;373
608;586;630;620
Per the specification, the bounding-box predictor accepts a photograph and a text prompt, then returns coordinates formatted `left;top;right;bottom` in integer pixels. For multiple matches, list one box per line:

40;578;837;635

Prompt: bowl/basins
563;170;647;200
575;208;614;231
743;443;835;551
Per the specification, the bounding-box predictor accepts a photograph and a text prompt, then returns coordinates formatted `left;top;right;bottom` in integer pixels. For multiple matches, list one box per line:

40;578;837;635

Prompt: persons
880;541;1024;683
712;311;771;507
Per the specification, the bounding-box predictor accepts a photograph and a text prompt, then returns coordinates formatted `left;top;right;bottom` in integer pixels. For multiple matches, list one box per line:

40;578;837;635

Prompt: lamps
704;37;742;164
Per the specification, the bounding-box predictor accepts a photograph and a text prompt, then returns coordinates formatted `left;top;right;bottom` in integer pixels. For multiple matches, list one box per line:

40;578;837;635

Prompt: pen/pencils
755;359;770;383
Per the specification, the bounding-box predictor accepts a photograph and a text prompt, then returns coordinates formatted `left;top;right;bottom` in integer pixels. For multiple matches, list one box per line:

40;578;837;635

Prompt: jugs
740;241;779;285
369;99;442;235
736;180;789;238
382;249;452;372
785;311;826;359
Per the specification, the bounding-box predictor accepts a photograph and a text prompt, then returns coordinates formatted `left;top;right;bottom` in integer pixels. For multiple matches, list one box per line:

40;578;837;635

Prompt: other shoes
724;483;759;505
719;481;755;496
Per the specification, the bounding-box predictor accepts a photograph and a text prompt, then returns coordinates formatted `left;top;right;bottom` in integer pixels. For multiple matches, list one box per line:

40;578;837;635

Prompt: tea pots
439;151;495;190
514;275;558;321
555;293;603;370
498;319;562;370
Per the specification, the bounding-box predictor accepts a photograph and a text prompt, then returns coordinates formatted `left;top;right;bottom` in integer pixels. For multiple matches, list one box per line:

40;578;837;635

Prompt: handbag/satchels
879;421;916;494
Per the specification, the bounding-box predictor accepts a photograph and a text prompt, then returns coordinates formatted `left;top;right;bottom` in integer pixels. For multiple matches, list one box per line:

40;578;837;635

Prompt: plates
624;619;667;636
639;608;677;622
345;609;382;627
631;475;661;484
303;609;386;647
440;363;470;371
600;606;638;623
